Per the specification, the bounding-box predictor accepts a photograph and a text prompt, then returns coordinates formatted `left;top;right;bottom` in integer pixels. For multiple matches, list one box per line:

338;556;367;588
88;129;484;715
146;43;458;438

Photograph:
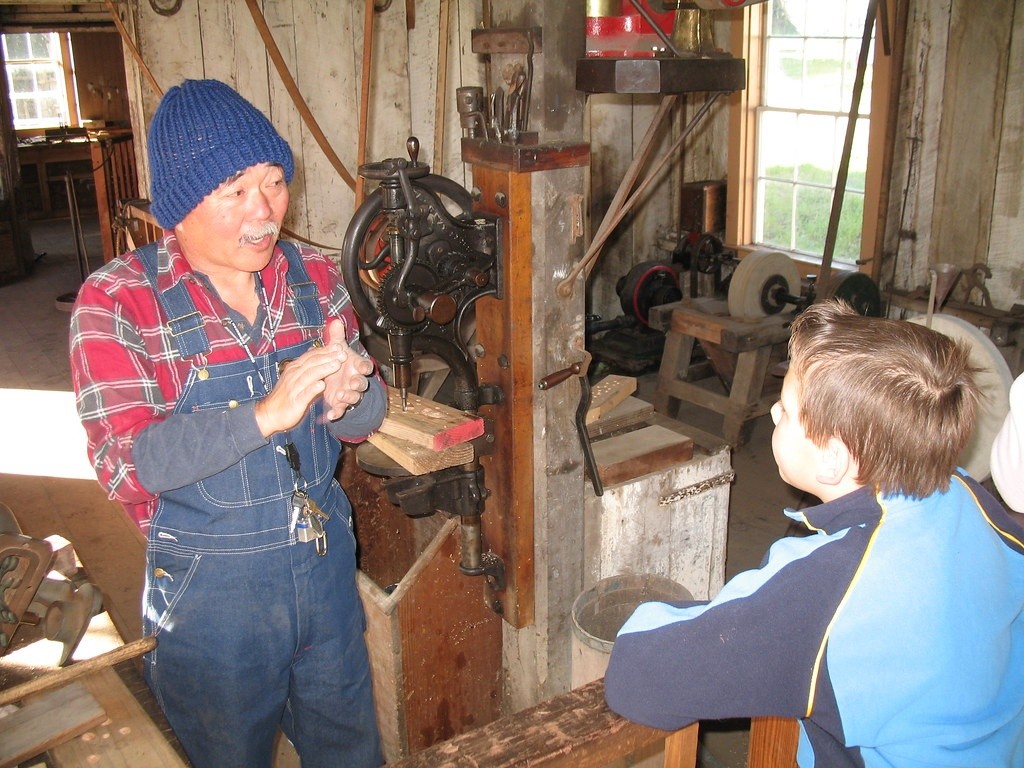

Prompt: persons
604;301;1023;767
68;80;389;767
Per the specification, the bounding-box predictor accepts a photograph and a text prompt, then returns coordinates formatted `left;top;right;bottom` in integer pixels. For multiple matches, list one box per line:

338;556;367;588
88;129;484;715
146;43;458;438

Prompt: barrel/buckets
571;572;695;689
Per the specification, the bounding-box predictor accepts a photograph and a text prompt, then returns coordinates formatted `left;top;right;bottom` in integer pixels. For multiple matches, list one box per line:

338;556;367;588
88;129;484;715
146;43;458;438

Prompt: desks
17;135;90;216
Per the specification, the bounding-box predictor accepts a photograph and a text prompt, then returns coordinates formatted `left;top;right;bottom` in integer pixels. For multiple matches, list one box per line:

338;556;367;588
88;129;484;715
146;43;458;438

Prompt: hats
149;79;294;230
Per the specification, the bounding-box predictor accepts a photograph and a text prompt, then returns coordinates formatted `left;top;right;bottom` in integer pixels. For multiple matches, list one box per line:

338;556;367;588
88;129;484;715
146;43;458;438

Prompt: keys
290;490;329;534
